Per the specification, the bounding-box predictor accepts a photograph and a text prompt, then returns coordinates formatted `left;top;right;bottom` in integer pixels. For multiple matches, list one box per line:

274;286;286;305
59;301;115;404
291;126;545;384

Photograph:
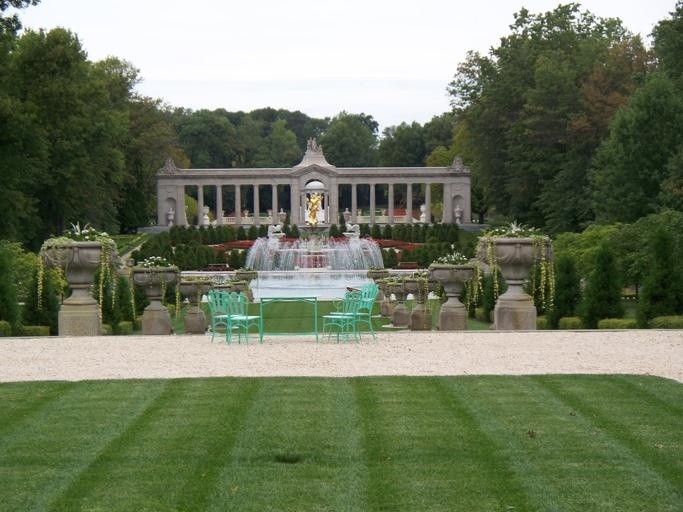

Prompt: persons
306;192;320;221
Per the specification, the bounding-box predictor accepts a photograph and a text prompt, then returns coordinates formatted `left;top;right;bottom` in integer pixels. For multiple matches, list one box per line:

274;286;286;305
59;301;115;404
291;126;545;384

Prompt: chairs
321;283;380;344
206;289;261;344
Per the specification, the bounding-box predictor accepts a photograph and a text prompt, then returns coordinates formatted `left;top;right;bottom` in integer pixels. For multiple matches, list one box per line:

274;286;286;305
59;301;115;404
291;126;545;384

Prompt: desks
259;296;318;344
